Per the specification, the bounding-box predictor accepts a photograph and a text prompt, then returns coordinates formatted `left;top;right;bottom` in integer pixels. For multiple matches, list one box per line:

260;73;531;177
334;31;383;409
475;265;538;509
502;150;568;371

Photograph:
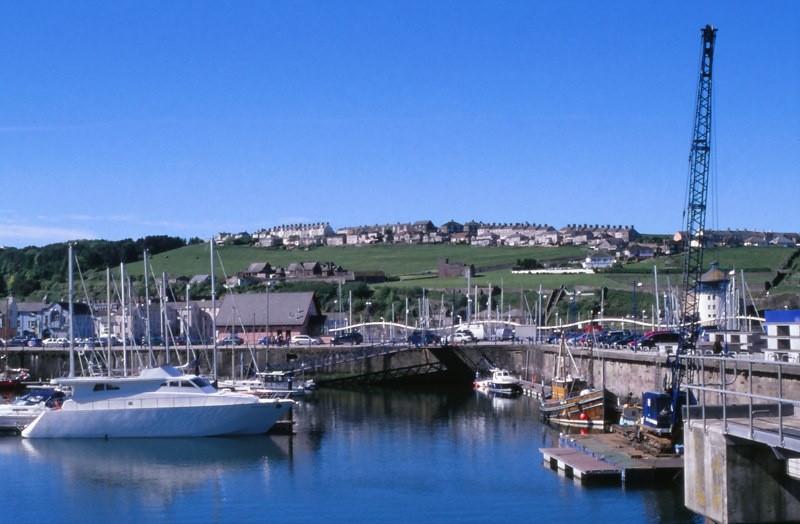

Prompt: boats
1;237;699;440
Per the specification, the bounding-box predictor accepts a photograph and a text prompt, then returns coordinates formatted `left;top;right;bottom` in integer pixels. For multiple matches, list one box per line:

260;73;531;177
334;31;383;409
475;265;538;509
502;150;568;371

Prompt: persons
271;333;291;346
713;340;722;354
